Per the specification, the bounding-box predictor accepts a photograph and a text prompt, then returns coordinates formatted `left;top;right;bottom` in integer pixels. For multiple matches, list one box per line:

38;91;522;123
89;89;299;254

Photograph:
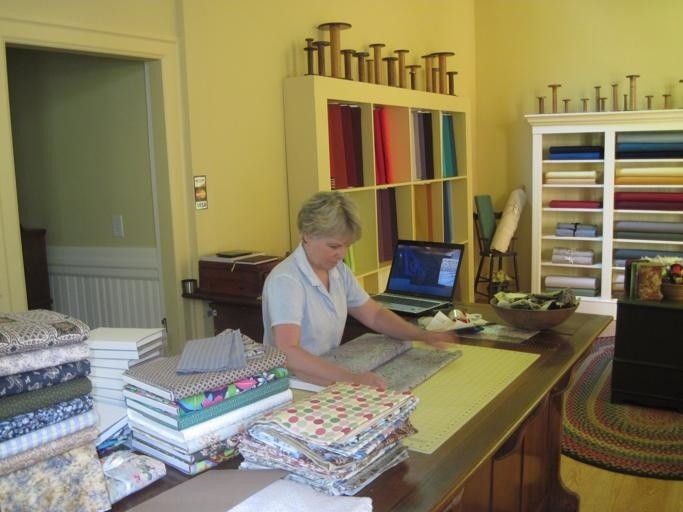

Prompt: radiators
46;245;163;331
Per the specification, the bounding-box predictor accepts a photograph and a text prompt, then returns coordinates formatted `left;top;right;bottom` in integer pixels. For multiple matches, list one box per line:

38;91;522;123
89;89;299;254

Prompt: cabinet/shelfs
612;296;682;414
524;109;683;319
283;76;475;304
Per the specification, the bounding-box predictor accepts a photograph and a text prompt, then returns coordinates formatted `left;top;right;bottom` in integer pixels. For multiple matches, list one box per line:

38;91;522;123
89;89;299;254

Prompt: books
81;321;295;480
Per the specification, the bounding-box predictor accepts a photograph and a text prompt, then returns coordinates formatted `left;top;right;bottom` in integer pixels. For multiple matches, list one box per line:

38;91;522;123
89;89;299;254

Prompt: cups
181;278;197;295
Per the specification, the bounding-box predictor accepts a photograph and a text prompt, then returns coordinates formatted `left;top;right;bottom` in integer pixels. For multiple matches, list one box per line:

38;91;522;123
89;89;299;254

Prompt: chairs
473;212;519;303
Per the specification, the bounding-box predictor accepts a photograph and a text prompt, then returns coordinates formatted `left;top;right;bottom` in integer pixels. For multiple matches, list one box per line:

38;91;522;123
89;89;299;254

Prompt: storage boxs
199;251;284;299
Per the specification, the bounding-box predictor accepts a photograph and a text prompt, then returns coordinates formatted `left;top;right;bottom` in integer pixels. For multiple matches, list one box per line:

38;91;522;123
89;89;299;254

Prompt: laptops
371;240;464;316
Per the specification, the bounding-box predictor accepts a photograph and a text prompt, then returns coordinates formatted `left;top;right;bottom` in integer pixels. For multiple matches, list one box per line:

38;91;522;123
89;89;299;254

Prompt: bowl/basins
660;283;682;302
487;295;578;329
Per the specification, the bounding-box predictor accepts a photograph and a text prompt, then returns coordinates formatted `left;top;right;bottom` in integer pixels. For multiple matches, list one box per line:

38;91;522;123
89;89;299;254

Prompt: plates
417;311;488;332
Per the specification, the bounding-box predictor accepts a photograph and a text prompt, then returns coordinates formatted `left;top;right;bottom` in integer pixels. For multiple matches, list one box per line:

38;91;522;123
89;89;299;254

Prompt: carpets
559;336;683;480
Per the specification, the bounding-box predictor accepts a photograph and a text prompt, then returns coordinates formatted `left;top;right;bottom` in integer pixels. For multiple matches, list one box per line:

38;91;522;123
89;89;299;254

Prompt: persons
258;190;460;395
